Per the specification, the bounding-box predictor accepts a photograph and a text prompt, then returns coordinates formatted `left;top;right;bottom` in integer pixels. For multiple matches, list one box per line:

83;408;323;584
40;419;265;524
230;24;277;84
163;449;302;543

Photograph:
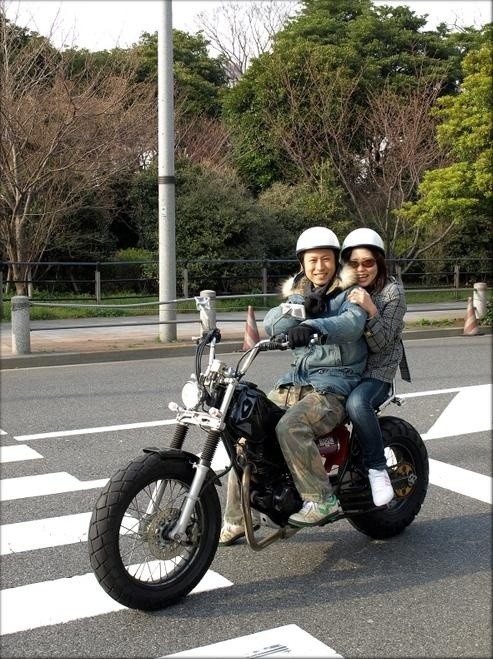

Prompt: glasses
349;259;376;269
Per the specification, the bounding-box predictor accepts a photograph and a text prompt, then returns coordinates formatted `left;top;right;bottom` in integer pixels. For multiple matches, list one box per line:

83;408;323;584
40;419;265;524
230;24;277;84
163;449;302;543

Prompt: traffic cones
458;297;485;336
237;305;267;351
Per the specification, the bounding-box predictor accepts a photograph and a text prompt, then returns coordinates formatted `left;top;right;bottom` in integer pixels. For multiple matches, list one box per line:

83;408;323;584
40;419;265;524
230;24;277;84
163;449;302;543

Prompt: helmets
340;226;386;258
294;226;342;258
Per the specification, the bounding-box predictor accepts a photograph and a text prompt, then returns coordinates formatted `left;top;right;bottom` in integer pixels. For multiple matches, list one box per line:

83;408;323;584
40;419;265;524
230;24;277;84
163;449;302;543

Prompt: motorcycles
87;296;429;612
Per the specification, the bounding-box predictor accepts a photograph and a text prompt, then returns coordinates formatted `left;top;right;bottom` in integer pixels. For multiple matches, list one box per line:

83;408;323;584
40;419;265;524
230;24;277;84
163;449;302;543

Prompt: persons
211;224;369;550
337;227;409;507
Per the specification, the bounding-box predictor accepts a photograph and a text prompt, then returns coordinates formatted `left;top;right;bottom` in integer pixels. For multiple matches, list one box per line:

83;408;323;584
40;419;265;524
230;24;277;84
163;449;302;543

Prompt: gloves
302;293;327;319
287;324;315;351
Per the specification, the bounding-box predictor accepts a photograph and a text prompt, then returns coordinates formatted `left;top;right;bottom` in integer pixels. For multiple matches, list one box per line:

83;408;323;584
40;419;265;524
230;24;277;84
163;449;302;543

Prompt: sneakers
366;467;396;507
217;516;263;546
286;494;344;527
